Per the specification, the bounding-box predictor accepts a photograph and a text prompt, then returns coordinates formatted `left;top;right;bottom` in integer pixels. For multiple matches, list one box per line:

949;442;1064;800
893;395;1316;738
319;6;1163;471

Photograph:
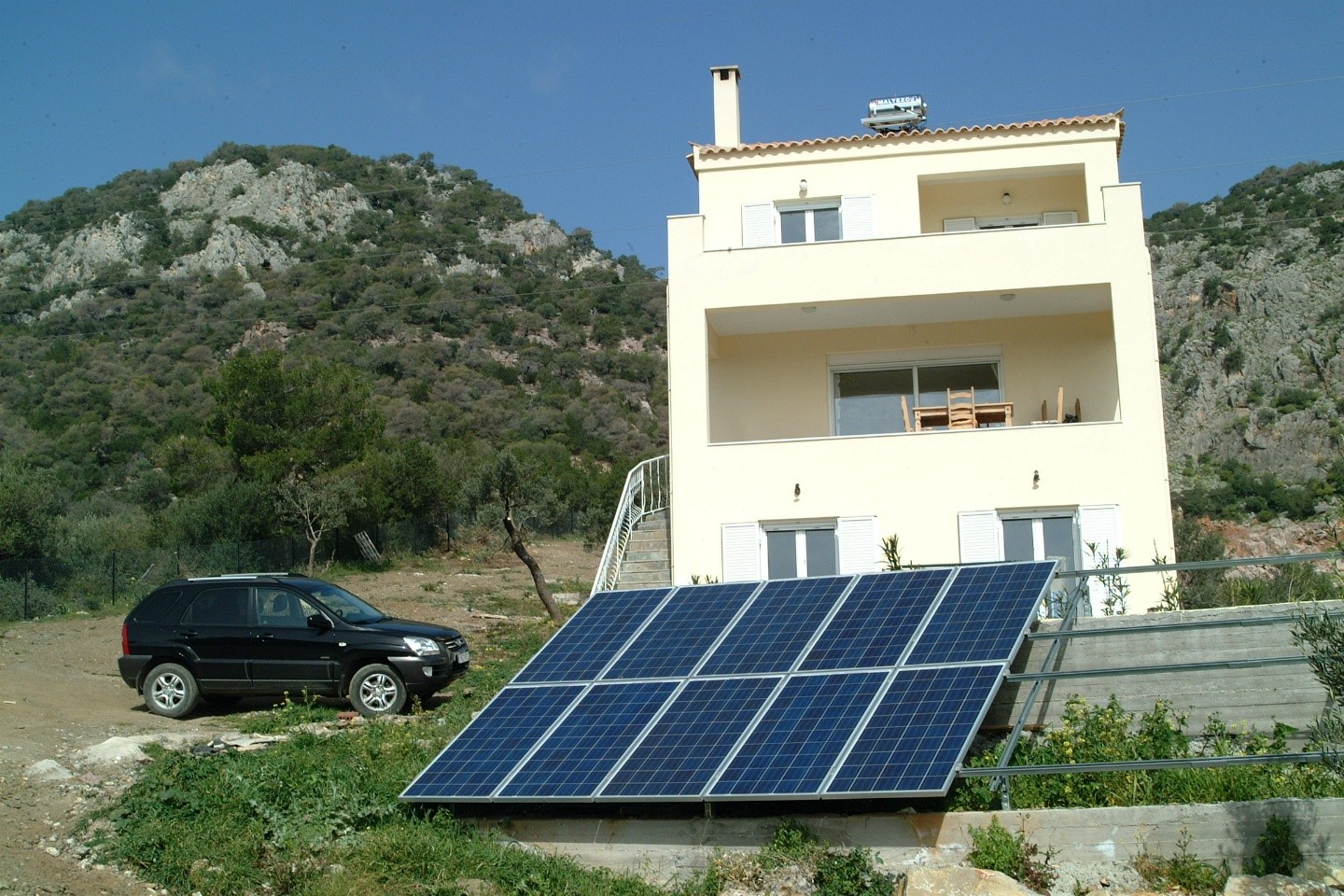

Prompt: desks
912;402;1016;431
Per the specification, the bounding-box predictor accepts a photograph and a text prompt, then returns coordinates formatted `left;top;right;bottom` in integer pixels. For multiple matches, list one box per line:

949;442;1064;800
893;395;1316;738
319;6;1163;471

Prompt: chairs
898;394;933;431
947;386;979;428
1029;387;1082;425
236;594;298;625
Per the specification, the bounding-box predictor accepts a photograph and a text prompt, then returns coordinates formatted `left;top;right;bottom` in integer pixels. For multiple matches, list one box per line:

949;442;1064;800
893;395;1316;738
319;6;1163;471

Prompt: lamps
798;177;808;194
1001;191;1014;205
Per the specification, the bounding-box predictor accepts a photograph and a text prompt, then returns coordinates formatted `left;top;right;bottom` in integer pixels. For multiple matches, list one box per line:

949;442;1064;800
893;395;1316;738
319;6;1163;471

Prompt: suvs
117;571;472;721
